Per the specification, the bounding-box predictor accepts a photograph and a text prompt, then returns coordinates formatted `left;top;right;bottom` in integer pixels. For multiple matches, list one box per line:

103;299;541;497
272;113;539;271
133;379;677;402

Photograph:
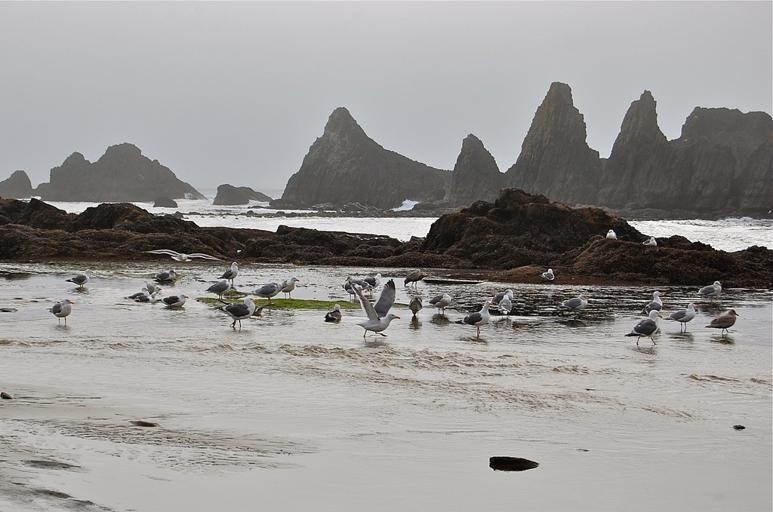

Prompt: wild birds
46;300;73;326
217;262;239;284
606;229;618;241
704;310;738;337
641;290;663;314
205;277;300;329
455;300;492;333
343;272;400;338
429;293;451;315
541;268;555;280
324;304;341;324
65;269;93;290
124;270;189;309
138;250;162;255
663;303;698;333
408;296;423;315
404;271;429;286
625;310;662;346
697;281;722;304
559;291;588;314
640;236;657;247
138;249;226;263
490;289;513;314
